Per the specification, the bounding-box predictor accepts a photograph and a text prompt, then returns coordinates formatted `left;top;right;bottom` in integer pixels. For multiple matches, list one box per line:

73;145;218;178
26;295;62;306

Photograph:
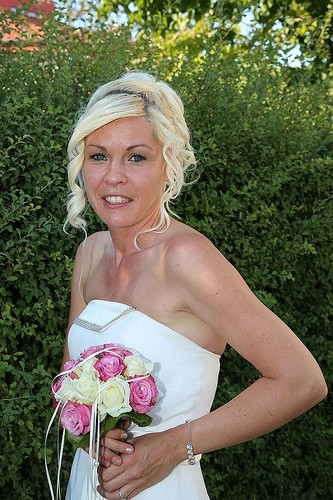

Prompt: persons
51;70;328;500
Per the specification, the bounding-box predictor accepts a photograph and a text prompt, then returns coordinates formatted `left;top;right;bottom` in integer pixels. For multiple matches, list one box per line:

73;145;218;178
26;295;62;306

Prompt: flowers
49;343;160;458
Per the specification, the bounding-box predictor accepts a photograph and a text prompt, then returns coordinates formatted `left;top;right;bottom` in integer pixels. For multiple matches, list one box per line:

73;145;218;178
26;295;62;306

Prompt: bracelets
185;419;203;467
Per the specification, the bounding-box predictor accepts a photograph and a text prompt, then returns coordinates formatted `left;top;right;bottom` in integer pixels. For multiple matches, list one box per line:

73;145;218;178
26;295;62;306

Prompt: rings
118;488;127;500
